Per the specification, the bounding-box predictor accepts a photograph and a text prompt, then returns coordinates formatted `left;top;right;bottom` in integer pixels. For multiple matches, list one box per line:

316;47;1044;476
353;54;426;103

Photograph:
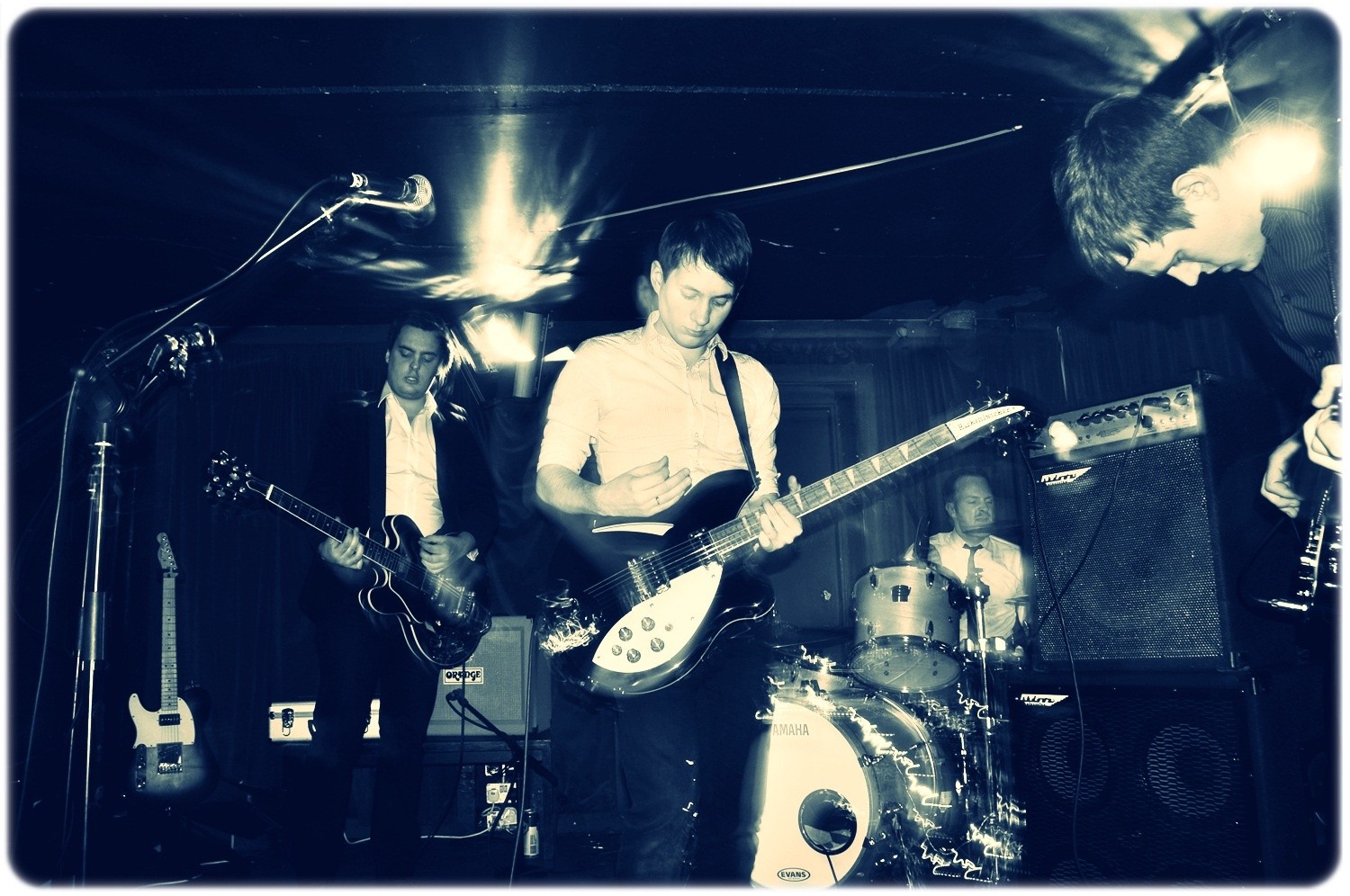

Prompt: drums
725;659;942;887
845;560;966;693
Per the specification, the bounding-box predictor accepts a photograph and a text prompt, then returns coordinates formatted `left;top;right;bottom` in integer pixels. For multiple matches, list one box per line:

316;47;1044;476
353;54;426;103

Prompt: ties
962;542;984;646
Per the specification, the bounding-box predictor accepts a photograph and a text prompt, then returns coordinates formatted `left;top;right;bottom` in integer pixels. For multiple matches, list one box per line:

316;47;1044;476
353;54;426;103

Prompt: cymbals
1006;593;1030;603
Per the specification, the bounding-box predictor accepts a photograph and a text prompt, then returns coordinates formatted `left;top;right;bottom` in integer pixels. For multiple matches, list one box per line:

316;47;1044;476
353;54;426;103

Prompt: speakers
1002;433;1333;887
427;615;538;738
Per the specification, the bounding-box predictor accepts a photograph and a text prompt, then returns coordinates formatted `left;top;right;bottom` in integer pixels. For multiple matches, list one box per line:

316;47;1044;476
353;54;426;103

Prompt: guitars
127;531;215;803
534;384;1035;714
1270;303;1341;626
201;448;493;670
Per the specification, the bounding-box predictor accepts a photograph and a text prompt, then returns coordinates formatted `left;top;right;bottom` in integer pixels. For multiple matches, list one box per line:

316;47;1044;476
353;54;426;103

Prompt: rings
656;498;662;507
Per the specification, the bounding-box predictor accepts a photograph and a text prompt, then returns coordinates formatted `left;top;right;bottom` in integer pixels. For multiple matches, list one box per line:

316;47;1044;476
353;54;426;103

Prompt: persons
904;468;1027;647
308;312;522;844
1052;92;1343;517
535;209;804;884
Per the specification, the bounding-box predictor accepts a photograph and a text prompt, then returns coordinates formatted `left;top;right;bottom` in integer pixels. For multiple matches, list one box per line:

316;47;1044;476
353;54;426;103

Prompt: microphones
332;172;433;211
447;688;464;700
